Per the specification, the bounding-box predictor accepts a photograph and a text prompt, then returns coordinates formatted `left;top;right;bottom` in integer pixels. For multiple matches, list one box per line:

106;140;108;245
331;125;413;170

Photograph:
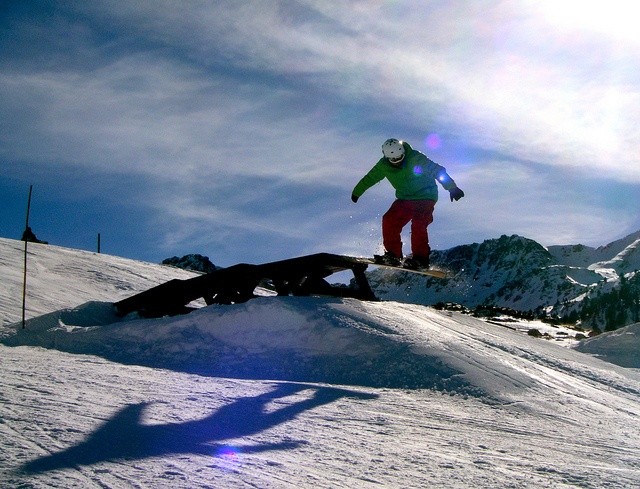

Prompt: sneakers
404;255;430;269
373;253;402;264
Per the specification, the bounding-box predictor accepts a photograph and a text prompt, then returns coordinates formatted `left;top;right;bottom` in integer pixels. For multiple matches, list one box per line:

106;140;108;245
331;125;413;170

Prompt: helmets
382;137;406;165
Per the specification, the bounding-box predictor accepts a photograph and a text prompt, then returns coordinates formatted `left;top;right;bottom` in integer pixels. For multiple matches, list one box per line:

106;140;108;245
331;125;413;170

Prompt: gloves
351;194;359;204
450;187;465;202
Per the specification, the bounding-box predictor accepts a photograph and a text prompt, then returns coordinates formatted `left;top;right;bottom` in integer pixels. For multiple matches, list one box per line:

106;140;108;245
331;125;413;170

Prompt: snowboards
349;252;450;280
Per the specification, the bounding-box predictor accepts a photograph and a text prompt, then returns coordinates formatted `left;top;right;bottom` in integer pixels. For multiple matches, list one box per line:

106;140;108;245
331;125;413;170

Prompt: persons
352;139;464;269
